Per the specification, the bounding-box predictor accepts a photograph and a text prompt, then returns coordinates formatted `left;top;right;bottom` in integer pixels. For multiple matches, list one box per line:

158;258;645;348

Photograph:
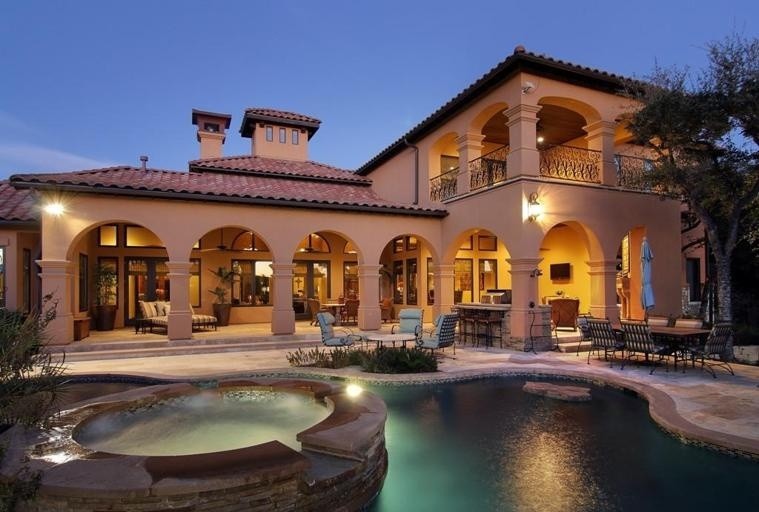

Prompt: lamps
527;191;543;223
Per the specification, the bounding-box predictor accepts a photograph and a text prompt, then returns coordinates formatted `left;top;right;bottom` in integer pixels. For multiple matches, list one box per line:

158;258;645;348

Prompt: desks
366;334;419;351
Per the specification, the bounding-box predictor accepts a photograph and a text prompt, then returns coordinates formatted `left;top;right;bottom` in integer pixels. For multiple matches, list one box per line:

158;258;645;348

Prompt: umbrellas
640;233;657;321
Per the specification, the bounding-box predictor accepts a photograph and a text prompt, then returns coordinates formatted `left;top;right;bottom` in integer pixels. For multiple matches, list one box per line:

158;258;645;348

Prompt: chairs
137;300;218;334
316;310;363;353
391;307;503;356
576;312;736;380
308;295;393;327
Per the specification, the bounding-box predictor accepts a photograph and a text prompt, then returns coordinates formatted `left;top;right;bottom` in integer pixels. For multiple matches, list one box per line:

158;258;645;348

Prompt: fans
198;226;245;254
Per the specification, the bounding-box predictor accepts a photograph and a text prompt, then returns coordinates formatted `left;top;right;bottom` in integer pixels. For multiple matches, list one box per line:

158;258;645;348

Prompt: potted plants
208;264;241;325
91;262;119;331
